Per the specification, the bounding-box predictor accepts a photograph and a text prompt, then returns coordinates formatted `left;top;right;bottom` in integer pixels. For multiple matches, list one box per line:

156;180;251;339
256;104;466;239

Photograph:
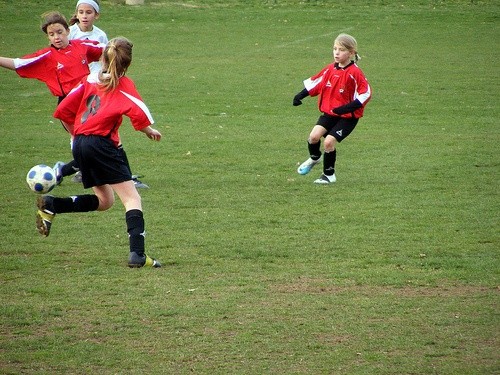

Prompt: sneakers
71;172;83;182
35;195;56;236
127;252;160;268
52;161;66;184
131;174;148;189
313;173;336;184
297;156;322;175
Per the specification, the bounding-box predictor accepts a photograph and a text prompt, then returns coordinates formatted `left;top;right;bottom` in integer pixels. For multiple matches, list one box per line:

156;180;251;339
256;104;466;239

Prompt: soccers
27;164;56;194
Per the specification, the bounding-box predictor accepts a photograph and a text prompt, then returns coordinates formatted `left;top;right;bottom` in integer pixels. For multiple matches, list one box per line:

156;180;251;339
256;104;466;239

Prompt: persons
293;33;372;183
67;0;108;82
36;37;163;267
0;12;150;188
